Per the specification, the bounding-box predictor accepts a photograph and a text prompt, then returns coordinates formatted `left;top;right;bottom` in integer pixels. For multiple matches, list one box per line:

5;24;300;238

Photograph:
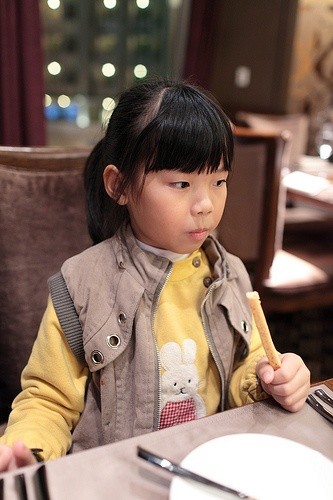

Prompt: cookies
246;291;281;370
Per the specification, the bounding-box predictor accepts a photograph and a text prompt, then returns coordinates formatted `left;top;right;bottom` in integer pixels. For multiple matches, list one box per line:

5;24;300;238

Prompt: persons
0;80;310;473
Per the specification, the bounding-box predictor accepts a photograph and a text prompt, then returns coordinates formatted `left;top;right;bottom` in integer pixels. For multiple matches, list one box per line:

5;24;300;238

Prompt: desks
0;378;333;500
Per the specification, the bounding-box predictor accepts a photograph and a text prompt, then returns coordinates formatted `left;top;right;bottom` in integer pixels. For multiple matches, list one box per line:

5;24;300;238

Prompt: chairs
242;114;329;231
0;151;94;426
216;128;333;379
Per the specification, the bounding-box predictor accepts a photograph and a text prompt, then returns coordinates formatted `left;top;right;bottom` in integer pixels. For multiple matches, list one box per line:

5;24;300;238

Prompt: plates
169;434;333;500
